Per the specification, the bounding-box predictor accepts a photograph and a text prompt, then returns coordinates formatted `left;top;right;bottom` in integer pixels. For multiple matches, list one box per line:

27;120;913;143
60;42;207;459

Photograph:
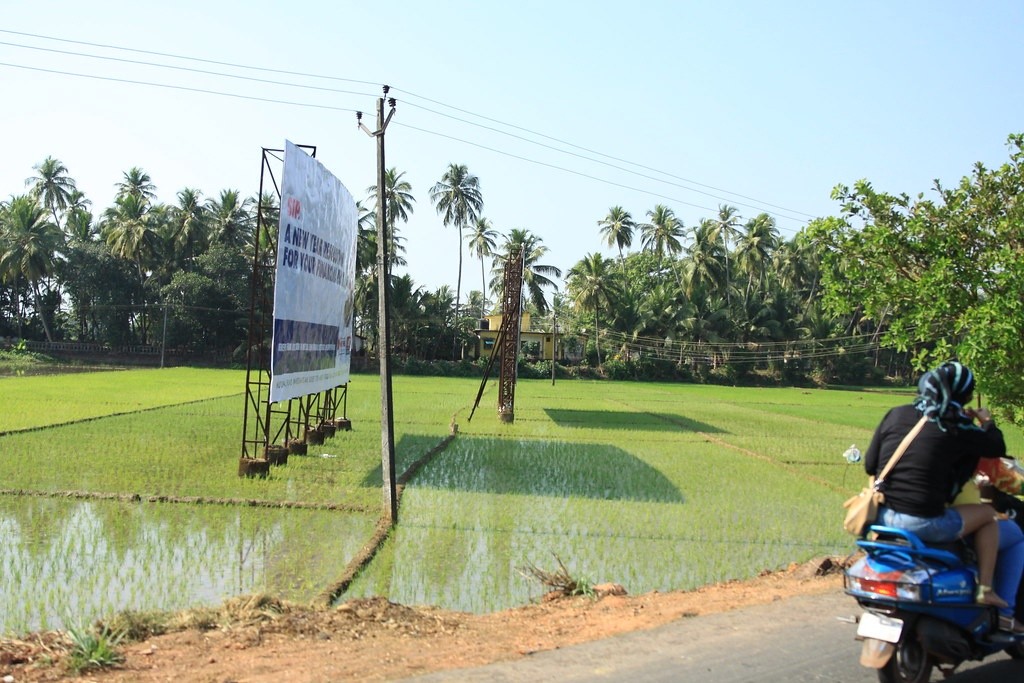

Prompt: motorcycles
840;452;1023;683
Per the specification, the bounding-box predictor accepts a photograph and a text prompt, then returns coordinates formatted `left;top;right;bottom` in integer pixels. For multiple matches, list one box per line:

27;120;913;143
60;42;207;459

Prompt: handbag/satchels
844;486;885;535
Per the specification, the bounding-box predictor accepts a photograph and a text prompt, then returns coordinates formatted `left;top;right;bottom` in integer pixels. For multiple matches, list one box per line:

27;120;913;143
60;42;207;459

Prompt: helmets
918;372;929;392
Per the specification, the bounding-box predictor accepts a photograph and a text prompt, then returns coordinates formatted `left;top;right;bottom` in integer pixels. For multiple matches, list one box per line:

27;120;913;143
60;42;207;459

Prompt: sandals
976;585;1009;607
999;615;1024;635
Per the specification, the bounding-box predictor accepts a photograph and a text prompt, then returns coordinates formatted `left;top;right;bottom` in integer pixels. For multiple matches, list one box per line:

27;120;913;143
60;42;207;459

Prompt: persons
863;361;1024;636
